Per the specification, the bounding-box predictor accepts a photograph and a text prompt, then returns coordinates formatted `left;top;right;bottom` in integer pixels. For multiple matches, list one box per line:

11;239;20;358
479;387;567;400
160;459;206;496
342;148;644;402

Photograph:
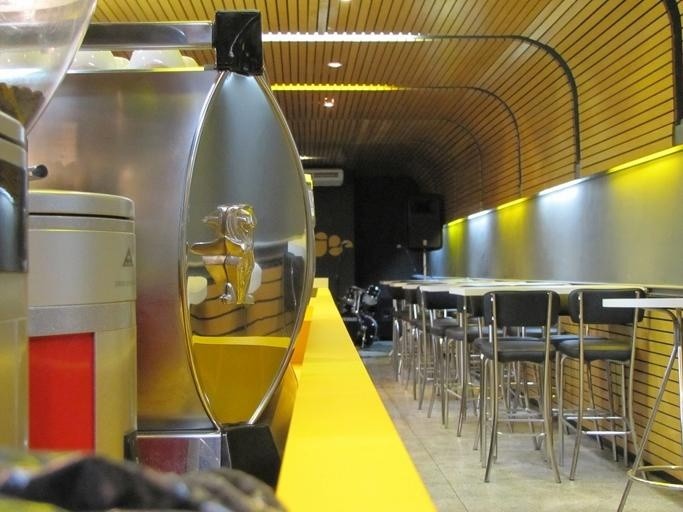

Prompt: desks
601;298;683;512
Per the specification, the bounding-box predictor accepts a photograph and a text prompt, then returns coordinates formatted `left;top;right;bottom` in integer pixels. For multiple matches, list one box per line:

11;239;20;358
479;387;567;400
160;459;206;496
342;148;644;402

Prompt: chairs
380;279;682;483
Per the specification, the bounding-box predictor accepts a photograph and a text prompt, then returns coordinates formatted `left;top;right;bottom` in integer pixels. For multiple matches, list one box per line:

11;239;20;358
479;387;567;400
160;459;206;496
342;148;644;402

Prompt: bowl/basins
72;44;200;70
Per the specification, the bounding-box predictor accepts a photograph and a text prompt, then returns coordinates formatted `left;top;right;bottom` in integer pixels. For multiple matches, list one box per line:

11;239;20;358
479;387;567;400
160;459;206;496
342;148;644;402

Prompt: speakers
407;195;442;251
